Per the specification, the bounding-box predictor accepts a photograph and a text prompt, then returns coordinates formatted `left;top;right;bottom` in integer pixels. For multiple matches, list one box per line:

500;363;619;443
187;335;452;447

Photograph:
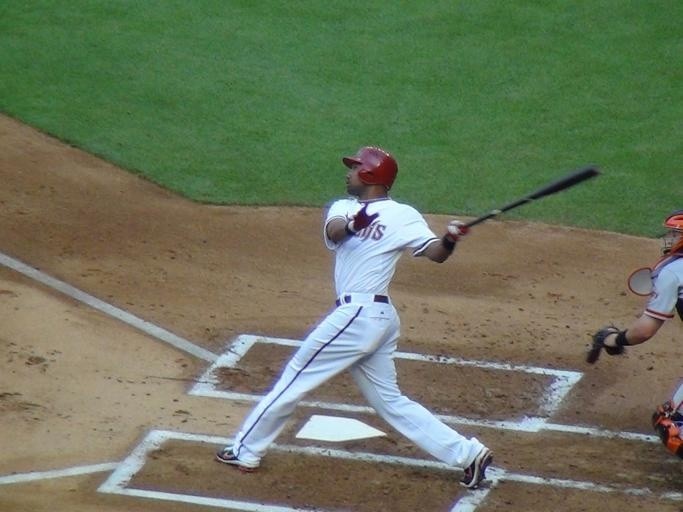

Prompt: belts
335;295;388;306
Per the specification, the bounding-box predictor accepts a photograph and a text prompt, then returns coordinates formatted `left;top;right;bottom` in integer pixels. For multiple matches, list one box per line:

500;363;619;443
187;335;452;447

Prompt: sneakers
460;446;493;488
216;450;259;472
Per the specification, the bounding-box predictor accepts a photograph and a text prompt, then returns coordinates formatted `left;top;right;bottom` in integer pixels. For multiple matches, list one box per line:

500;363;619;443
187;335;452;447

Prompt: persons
593;211;683;459
217;147;494;490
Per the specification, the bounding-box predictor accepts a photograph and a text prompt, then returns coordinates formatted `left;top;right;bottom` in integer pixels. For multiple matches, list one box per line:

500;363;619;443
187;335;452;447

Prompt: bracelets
442;238;456;249
615;329;630;347
344;222;355;235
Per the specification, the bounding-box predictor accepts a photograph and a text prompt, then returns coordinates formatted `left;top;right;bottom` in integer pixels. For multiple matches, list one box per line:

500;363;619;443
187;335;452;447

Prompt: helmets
659;210;683;255
343;147;397;190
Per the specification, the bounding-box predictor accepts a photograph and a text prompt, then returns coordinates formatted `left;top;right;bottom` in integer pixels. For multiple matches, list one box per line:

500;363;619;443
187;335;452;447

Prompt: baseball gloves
585;325;628;364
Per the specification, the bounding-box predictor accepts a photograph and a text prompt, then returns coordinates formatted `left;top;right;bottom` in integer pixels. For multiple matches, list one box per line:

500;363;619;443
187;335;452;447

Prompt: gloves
446;221;468;241
348;202;377;233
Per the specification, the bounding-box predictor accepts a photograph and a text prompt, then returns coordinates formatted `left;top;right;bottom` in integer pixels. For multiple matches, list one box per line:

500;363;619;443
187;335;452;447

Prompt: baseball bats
460;165;604;229
